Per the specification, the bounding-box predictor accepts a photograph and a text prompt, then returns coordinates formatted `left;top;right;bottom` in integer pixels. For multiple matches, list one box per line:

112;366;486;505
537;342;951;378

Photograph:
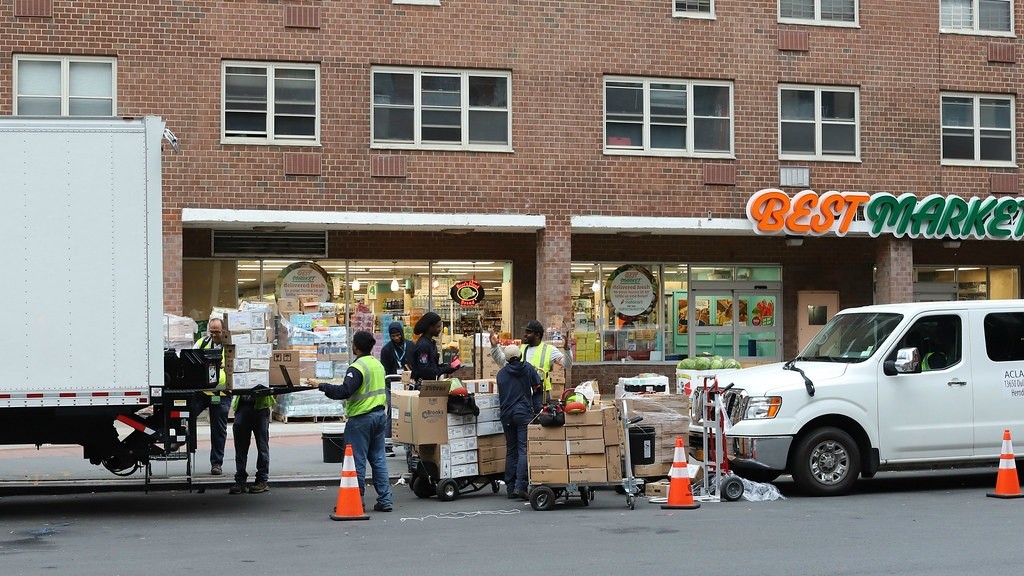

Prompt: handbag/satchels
447;393;480;416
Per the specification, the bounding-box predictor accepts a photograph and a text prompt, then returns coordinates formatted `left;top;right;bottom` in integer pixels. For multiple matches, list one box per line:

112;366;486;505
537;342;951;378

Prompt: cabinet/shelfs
382;275;502;335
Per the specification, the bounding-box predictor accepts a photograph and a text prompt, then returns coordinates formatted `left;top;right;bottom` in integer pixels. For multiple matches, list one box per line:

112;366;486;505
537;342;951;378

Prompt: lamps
352;261;360;291
592;264;600;293
390;261;400;292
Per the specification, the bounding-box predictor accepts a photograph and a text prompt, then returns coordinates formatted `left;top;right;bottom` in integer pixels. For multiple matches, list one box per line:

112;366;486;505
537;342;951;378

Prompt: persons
305;331;393;512
183;317;231;474
380;321;416;457
229;394;275;494
906;332;947;372
495;344;542;501
489;319;573;404
406;311;459;472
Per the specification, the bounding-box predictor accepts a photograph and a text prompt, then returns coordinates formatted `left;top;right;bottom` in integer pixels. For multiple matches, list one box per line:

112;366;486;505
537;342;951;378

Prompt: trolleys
527;399;644;511
403;443;506;501
701;376;744;502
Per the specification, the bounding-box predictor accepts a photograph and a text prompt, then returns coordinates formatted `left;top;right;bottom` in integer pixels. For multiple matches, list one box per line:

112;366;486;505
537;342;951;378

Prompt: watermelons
676;354;742;369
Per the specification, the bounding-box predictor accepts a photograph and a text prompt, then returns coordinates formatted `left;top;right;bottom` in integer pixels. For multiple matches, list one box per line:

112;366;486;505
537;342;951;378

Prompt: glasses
208;330;223;334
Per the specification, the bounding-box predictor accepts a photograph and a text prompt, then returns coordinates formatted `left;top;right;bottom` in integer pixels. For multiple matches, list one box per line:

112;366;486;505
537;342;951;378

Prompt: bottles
454;298;502;337
382;296;450;319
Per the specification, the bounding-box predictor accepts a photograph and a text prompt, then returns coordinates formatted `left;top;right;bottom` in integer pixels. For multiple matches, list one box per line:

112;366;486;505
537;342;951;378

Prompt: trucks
0;115;179;478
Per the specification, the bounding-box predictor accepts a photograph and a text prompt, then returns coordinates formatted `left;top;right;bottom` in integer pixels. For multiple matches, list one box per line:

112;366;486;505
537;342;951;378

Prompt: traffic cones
329;445;370;520
660;436;701;510
985;429;1024;499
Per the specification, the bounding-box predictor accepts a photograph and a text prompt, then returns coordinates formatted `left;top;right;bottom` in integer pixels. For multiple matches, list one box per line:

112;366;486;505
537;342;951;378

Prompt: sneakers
230;480;247;494
508;489;529;501
374;504;392;512
249;481;269;492
211;464;222;475
170;442;186;451
385;447;395;457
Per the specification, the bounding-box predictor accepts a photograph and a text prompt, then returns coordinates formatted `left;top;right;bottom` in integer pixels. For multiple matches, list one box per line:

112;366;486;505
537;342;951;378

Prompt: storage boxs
162;295;694;497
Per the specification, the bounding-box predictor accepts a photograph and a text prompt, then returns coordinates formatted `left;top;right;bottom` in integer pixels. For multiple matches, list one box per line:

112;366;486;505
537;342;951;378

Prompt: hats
354;331;376;351
504;345;521;361
521;320;544;333
414;312;441;335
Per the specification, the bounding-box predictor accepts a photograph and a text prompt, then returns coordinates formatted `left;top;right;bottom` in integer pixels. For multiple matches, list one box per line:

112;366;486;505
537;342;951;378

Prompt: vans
687;298;1024;497
